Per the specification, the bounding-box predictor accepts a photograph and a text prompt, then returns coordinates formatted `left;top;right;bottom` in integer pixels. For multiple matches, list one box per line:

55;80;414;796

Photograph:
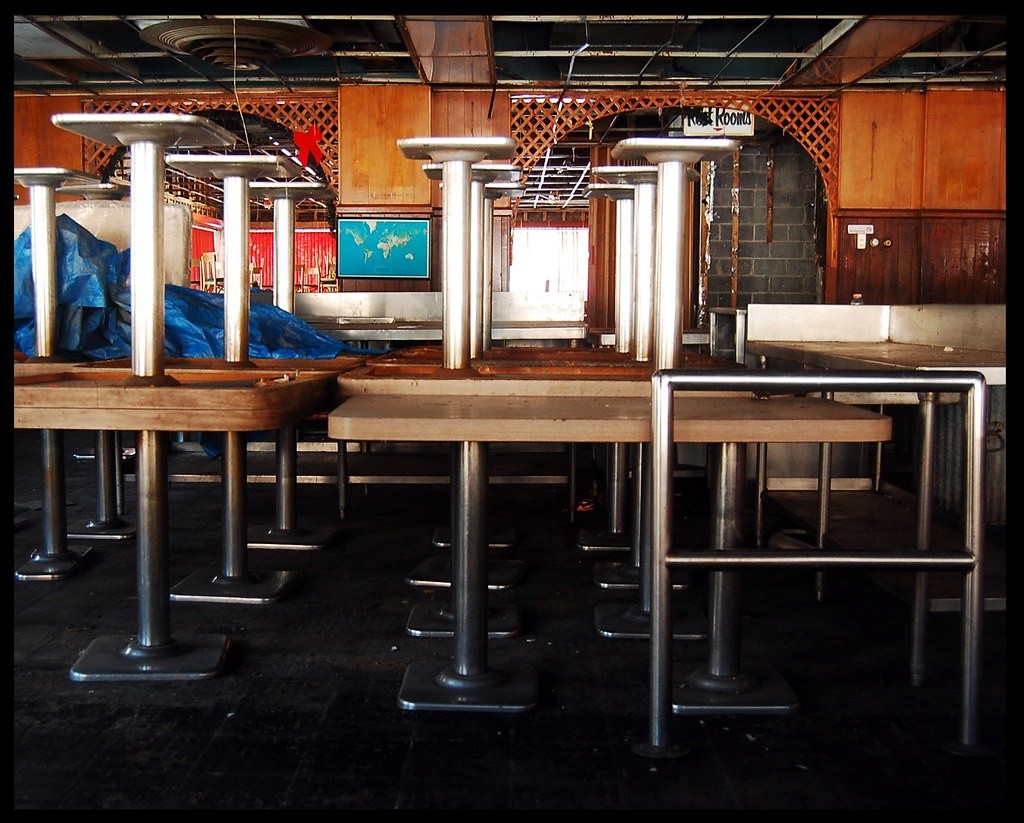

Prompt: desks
15;109;1005;720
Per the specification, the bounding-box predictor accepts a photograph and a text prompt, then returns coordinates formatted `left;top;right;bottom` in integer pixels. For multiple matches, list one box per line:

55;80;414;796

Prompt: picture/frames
335;216;433;281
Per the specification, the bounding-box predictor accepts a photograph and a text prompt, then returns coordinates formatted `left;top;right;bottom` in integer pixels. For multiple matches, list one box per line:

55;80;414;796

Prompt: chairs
188;251;341;294
